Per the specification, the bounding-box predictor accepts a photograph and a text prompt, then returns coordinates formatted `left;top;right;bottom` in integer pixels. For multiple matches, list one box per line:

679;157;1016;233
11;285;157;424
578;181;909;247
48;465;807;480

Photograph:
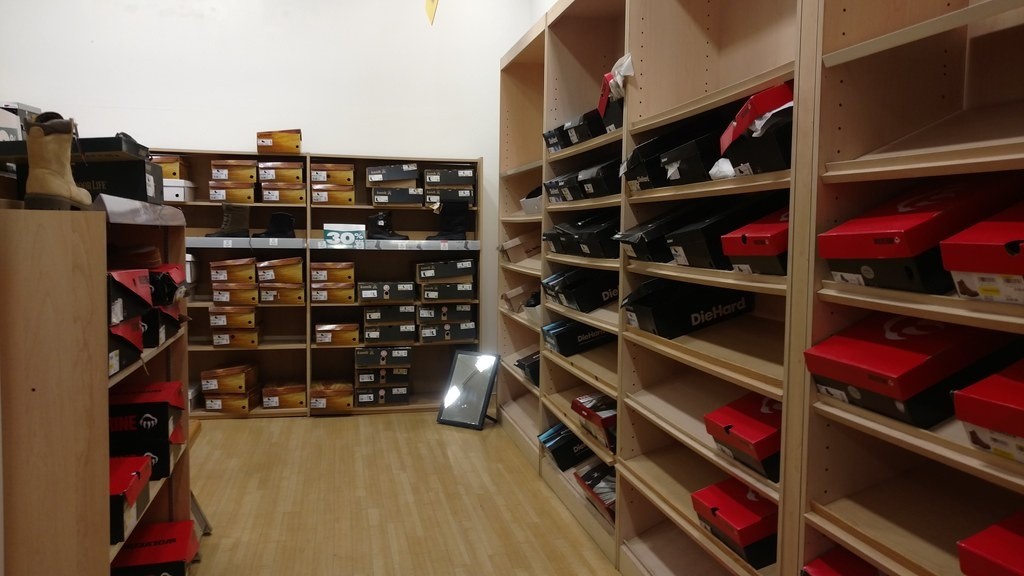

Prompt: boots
21;114;95;213
425;198;470;242
368;211;411;243
252;211;298;240
205;202;251;239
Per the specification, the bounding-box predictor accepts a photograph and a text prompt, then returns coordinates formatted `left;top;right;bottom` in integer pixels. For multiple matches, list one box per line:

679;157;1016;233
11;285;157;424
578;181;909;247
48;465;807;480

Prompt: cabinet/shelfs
146;147;484;421
496;0;1024;575
1;198;212;576
